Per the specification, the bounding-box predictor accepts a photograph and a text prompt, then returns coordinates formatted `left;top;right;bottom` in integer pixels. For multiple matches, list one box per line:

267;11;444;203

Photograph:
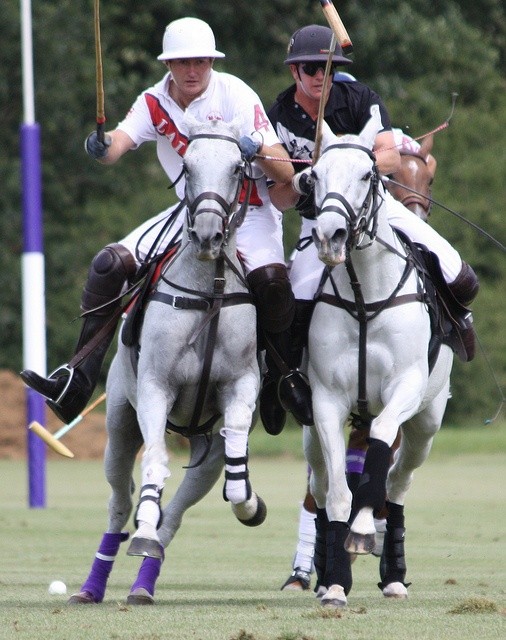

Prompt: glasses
302;63;336;75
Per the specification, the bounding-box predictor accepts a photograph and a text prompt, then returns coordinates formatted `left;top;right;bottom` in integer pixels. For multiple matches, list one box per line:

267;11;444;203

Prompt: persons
257;22;479;435
20;16;315;428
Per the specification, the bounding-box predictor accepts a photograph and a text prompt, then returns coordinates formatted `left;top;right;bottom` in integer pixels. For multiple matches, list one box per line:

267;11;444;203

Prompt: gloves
83;130;111;159
292;166;315;196
238;135;260;162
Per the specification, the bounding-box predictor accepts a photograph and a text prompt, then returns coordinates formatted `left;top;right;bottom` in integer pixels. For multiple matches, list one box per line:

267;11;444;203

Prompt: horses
280;113;456;605
279;133;440;592
65;108;269;607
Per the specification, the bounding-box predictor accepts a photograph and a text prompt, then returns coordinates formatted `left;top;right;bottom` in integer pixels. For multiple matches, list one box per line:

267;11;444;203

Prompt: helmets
157;17;225;60
282;24;354;66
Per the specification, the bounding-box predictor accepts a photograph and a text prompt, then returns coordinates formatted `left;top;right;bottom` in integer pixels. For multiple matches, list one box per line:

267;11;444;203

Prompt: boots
20;305;123;425
263;323;315;427
259;299;315;435
